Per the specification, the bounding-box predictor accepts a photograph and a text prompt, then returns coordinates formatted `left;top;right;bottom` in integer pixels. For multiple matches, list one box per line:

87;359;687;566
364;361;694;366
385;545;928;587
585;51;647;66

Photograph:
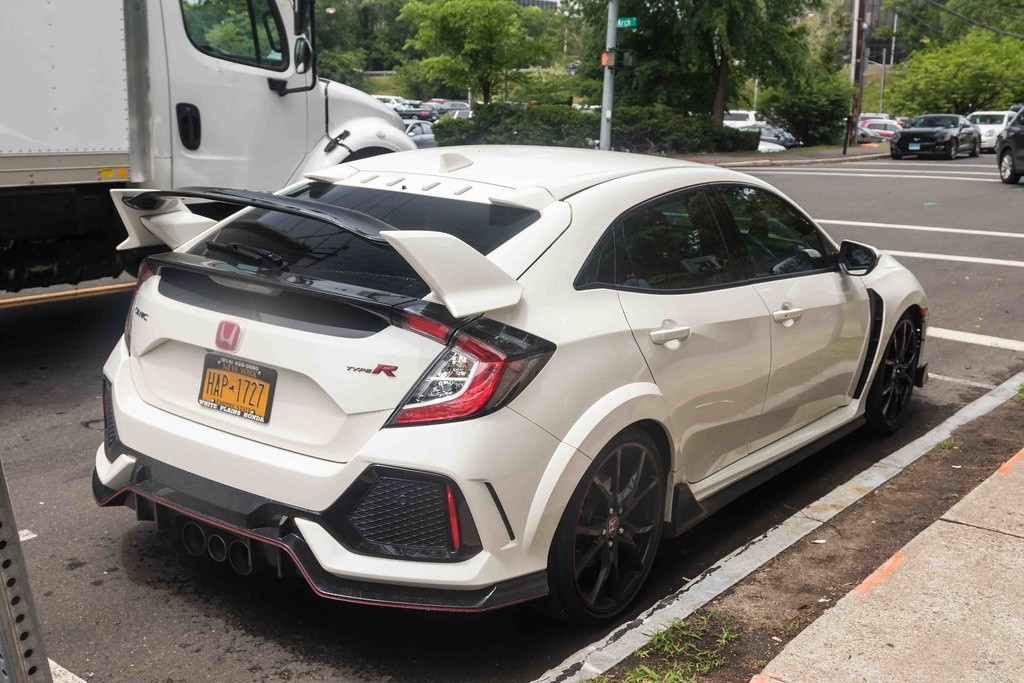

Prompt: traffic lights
602;48;619;67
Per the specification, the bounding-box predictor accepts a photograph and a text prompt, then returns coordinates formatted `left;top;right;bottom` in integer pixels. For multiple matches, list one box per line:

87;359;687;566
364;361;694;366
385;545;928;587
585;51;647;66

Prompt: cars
89;144;931;637
994;106;1024;184
965;110;1018;154
370;93;602;151
890;113;982;160
718;109;802;154
842;113;915;144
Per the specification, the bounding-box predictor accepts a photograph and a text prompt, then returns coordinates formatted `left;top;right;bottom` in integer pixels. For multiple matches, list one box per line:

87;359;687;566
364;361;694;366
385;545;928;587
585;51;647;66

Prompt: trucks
1;0;421;306
